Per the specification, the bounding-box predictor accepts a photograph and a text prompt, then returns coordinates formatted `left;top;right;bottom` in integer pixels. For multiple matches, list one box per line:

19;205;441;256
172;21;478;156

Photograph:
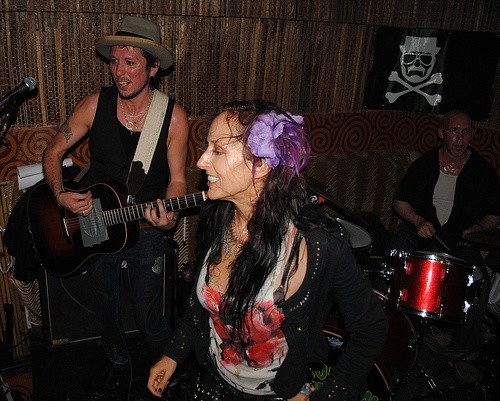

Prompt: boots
90;359;143;401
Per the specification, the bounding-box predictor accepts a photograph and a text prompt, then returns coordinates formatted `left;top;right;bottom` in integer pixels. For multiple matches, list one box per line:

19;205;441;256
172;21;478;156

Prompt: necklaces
224;220;245;260
119;90;154;131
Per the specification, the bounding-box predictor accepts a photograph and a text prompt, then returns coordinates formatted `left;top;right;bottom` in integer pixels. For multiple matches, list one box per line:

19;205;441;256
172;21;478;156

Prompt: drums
333;217;373;262
396;248;470;325
322;272;418;394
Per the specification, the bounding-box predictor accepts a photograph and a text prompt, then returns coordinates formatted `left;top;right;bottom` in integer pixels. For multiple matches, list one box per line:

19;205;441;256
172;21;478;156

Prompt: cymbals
465;227;500;248
307;176;350;213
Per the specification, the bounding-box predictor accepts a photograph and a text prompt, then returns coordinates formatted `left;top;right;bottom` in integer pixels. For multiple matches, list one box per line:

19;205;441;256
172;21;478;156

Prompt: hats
94;15;173;71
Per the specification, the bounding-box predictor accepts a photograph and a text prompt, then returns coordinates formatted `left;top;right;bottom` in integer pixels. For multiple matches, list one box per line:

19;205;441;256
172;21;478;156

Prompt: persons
392;109;500;347
38;16;187;366
148;100;391;400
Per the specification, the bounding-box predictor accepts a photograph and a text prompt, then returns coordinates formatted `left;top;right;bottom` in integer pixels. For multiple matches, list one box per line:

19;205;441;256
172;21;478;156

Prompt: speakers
37;239;177;351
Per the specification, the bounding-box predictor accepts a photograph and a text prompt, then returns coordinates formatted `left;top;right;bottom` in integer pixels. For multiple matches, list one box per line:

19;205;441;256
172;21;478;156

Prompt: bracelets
55;190;64;199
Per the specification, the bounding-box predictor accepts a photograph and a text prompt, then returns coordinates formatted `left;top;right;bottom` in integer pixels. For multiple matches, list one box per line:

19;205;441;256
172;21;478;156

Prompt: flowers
246;110;310;176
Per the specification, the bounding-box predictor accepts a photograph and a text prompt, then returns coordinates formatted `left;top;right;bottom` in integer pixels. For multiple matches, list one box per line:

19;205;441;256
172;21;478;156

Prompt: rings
82;211;84;217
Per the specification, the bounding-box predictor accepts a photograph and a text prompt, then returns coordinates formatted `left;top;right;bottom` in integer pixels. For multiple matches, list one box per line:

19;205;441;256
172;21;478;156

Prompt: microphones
0;77;37;112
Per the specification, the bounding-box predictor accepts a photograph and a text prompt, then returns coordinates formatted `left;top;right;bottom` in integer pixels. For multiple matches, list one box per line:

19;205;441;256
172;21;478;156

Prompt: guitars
26;177;211;279
